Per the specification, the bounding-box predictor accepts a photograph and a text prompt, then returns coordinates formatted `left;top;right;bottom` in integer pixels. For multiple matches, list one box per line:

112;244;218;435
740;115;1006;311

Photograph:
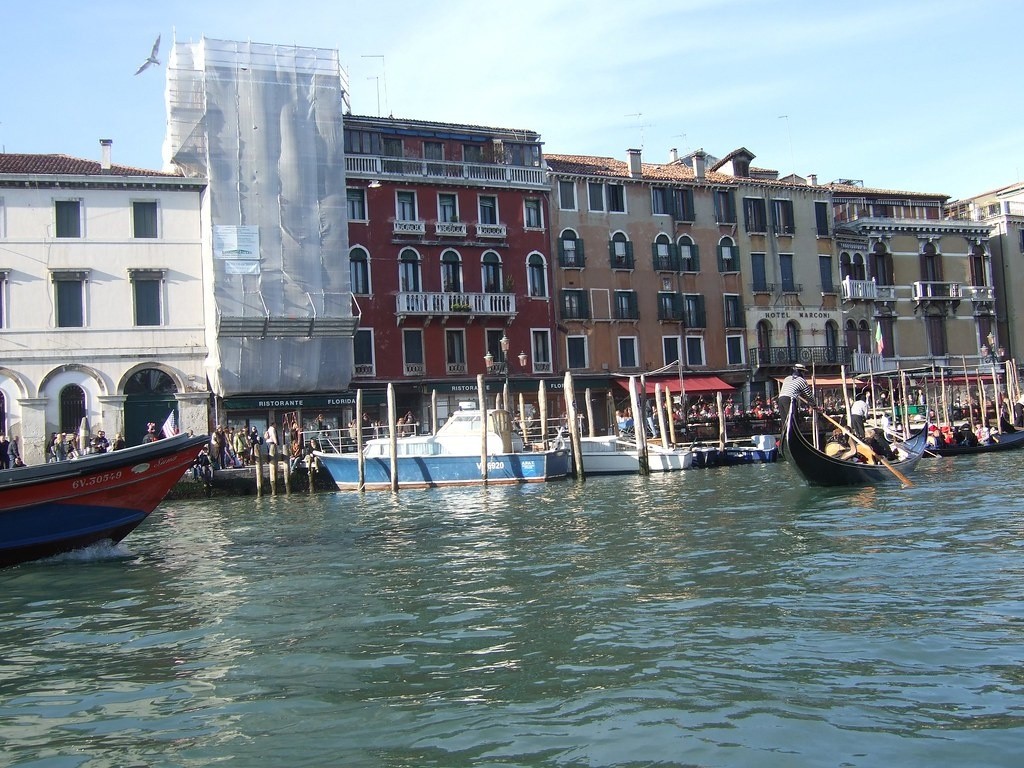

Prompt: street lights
484;334;528;422
981;331;1005;368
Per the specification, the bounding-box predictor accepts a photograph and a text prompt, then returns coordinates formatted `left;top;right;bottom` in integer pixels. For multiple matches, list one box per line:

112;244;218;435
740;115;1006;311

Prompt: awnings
773;375;865;384
615;376;735;395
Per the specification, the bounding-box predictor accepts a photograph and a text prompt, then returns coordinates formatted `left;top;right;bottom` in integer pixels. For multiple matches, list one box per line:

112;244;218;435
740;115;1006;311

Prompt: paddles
797;395;915;488
863;420;941;458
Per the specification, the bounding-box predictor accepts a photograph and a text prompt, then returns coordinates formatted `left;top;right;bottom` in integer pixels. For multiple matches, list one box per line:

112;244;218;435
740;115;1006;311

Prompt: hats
792;364;807;371
216;425;222;429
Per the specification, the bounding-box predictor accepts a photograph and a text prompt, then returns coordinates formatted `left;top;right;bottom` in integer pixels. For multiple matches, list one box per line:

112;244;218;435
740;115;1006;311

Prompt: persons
851;395;868;435
908;388;1024;447
514;411;521;423
189;422;320;487
0;433;25;468
864;391;872;408
775;363;816;447
314;414;325;438
345;410;417;450
45;430;126;464
143;422;159;444
827;427;893;460
614;395;847;439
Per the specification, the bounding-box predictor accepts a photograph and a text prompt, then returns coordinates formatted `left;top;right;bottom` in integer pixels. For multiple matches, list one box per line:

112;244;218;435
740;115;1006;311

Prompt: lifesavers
552;438;566;449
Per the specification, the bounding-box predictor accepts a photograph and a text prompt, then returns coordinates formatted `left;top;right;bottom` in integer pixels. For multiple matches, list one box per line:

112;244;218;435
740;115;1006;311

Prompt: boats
684;437;779;470
526;434;695;474
914;428;1024;456
0;433;213;567
782;397;930;485
312;406;570;492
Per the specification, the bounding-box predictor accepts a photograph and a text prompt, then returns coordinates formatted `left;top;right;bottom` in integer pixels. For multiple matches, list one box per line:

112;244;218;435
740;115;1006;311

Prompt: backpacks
264;426;275;439
253;432;263;445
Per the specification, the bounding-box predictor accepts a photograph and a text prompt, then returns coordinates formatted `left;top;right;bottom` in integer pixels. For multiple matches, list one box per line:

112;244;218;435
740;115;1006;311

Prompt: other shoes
209;480;212;486
307;471;311;474
194;480;199;483
203;481;207;487
316;470;320;473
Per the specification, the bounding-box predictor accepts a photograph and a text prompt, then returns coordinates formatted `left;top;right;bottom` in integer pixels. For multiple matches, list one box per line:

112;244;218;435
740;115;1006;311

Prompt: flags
163;412;176;438
876;324;884;354
